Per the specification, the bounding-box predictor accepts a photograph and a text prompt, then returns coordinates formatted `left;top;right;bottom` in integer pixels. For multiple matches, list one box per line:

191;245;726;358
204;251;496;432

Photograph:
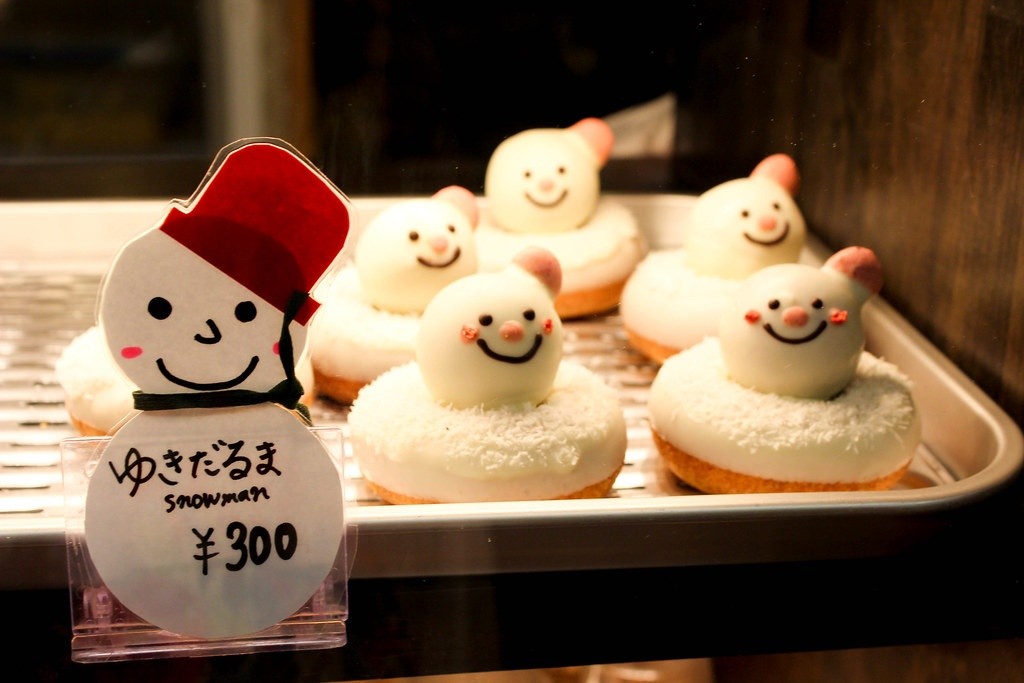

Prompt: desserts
50;119;919;504
